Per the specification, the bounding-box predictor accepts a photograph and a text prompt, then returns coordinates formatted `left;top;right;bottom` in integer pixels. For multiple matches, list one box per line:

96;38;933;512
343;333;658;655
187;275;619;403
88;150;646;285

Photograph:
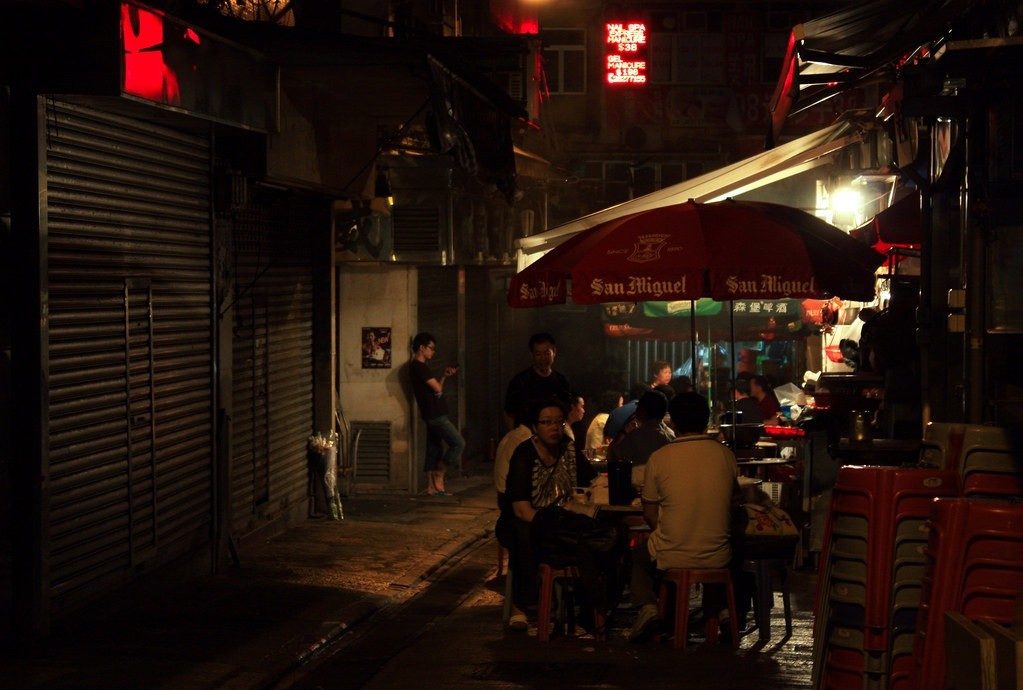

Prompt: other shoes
718;608;732;638
629;608;662;641
508;613;529;628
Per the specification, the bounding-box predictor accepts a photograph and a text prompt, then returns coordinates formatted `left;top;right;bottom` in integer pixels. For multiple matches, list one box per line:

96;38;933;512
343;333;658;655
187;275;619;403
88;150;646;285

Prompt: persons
362;332;380;354
502;332;572;430
841;322;923;466
411;332;465;496
494;359;778;642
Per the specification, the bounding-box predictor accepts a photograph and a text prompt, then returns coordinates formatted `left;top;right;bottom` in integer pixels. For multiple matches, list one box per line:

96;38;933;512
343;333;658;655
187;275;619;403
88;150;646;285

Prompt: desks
738;458;797;481
599;505;644;515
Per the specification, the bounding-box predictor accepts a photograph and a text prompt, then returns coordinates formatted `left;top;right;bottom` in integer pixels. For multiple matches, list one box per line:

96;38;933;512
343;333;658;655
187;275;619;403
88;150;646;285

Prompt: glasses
426;345;435;351
535;418;566;427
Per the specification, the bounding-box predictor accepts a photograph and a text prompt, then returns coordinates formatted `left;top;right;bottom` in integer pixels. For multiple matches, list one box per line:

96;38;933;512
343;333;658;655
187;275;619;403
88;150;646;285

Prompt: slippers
425;491;445;497
440;490;453;497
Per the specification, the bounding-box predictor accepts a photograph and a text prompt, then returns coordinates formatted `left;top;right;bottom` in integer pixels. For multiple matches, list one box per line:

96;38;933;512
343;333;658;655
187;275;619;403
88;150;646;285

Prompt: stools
497;542;606;643
654;558;792;651
811;422;1023;690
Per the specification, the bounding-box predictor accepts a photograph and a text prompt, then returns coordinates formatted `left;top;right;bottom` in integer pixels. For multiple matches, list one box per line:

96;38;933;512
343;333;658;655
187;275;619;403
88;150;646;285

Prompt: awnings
514;117;867;275
765;0;974;148
855;188;924;266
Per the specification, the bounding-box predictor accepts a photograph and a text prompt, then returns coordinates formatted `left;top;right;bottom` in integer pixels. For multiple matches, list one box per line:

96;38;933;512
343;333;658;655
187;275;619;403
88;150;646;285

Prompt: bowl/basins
825;345;844;362
834;307;860;325
719;422;765;447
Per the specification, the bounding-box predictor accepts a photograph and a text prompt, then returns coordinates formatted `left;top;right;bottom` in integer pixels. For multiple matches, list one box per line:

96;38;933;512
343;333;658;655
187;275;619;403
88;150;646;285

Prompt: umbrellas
722;198;888;440
507;195;877;392
599;301;837;425
